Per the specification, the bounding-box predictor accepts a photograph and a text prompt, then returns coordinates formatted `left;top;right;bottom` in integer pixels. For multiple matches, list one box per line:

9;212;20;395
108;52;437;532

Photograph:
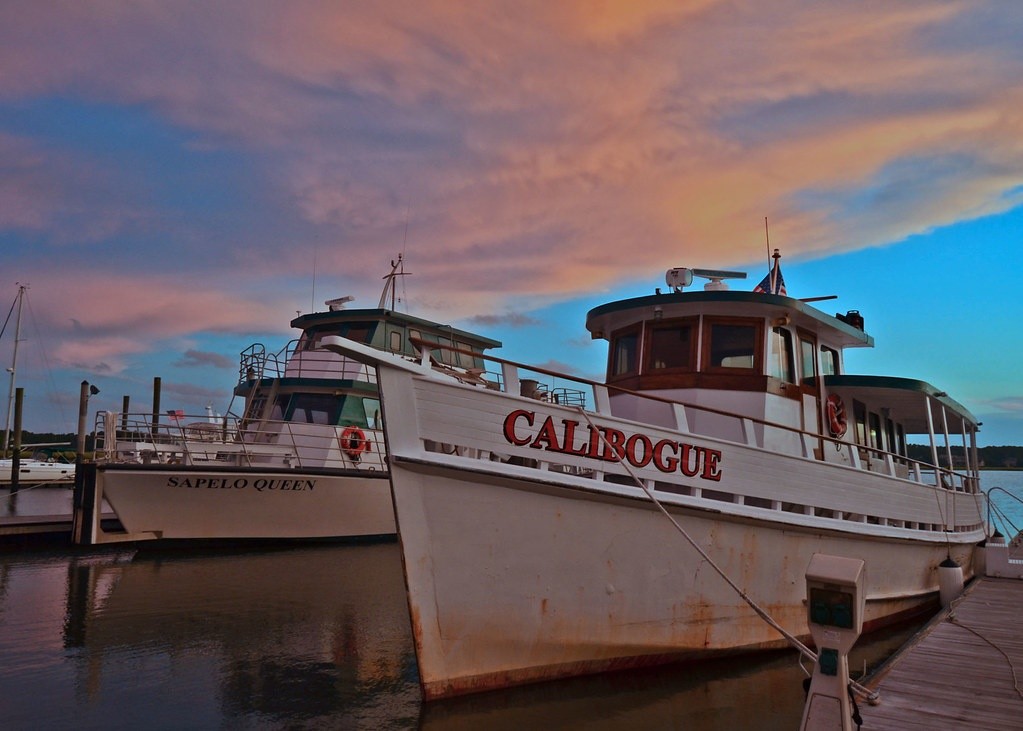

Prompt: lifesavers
826;394;847;435
340;426;366;455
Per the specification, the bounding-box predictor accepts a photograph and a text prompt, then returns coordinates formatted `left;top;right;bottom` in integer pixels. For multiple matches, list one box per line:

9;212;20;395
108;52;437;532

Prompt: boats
92;248;588;548
0;458;76;485
321;213;999;709
